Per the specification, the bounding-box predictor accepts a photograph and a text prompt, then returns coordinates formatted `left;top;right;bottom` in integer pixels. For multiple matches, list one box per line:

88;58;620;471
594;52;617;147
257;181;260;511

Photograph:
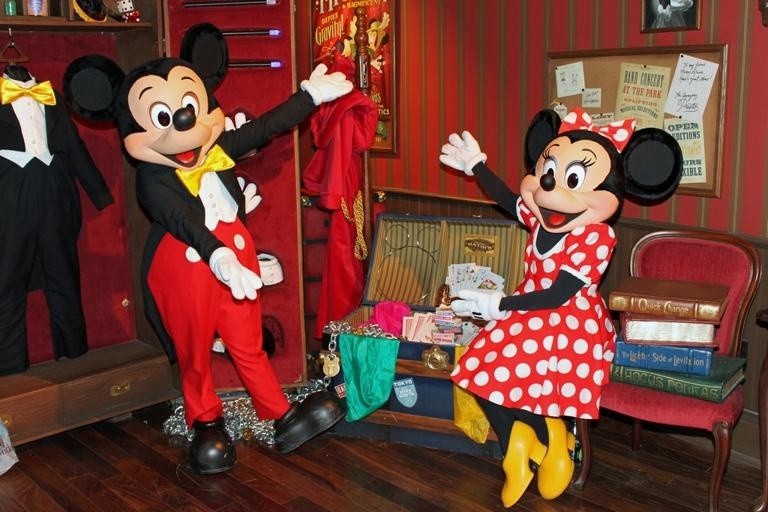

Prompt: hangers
1;34;29;68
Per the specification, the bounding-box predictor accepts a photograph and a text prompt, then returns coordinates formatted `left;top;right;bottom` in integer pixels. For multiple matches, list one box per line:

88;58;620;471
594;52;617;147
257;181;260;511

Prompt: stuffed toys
439;110;684;508
62;24;354;474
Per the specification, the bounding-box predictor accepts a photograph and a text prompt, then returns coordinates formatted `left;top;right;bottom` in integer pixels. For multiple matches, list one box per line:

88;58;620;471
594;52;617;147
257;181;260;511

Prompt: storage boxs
319;211;532;463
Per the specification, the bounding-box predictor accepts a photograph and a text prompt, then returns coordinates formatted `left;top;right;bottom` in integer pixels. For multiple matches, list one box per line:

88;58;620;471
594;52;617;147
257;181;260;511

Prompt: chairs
577;230;763;512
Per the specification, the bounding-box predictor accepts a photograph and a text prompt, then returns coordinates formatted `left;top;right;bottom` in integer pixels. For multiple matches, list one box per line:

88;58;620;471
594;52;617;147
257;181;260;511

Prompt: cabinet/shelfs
0;0;308;447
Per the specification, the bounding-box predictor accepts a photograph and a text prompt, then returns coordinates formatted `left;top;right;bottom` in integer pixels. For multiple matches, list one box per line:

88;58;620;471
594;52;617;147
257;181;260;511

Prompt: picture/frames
640;0;702;33
307;0;399;158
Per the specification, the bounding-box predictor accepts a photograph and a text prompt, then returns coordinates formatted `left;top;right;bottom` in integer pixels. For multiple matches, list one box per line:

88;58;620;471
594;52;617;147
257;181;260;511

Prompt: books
609;279;746;403
